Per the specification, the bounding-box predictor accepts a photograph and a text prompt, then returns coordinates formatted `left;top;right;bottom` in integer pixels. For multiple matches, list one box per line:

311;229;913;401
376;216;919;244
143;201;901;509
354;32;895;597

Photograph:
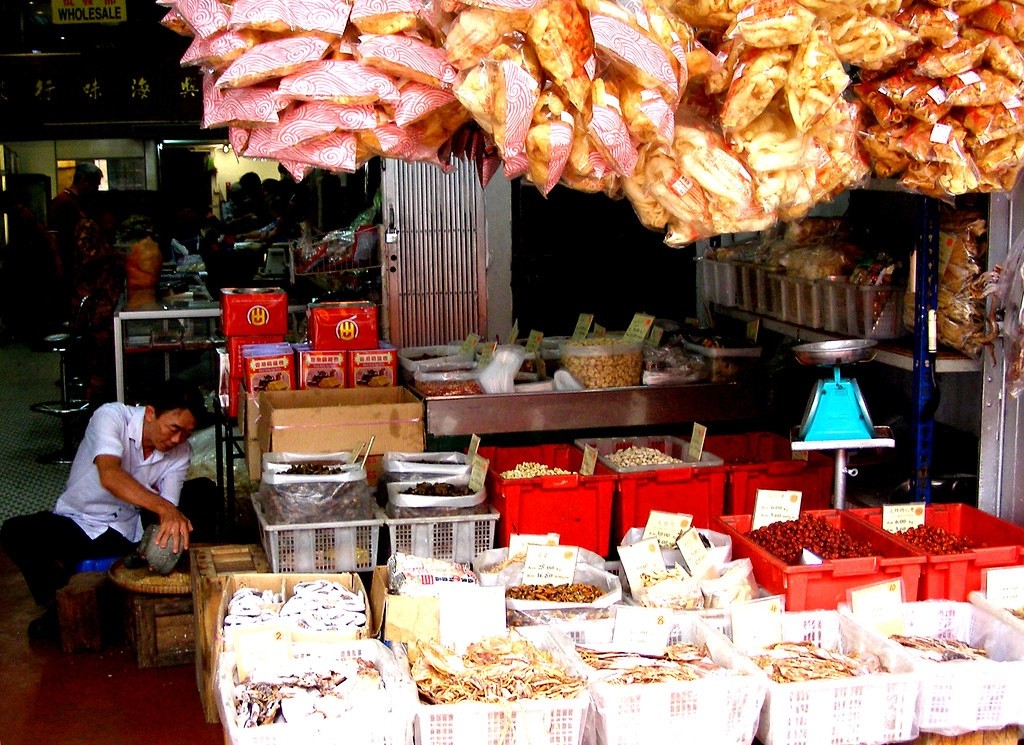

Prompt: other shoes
26;609;67;645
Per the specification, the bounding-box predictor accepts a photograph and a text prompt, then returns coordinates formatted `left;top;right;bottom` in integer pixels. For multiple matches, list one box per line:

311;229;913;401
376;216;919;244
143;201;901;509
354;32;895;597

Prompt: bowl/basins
139;525;184;575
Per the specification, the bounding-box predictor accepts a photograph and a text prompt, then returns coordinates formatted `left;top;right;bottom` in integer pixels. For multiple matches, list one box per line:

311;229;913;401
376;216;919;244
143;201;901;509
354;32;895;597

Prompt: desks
110;299;309;403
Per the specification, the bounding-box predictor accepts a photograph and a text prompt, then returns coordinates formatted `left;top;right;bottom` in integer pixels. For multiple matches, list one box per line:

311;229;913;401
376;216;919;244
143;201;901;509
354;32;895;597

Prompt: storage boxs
220;287;288;335
124;591;198;671
407;381;746;436
293;344;307;373
348;344;398;388
244;346;297;392
213;486;1024;745
237;383;261;482
241;342;291;385
259;386;427;484
703;431;836;515
222;369;243;416
694;254;907;340
188;543;273;723
297;348;347;389
574;435;732;545
466;442;619;560
225;335;285;377
307;300;380;350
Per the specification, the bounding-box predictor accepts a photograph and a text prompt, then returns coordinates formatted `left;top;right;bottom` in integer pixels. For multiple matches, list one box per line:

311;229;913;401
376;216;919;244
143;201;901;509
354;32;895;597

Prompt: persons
0;379;230;642
47;164;103;281
195;192;289;287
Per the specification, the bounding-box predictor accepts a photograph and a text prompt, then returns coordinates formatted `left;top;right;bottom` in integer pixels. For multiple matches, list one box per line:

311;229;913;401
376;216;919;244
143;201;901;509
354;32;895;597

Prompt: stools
77;554;120;575
34;330;99;466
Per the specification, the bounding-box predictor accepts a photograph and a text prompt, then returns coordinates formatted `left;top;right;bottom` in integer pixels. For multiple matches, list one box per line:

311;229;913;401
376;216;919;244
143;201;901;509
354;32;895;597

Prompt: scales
790;335;880;441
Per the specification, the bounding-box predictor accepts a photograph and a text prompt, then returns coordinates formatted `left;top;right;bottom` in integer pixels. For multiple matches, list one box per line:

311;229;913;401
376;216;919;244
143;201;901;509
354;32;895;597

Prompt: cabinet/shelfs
118;274;218;378
692;174;1024;526
